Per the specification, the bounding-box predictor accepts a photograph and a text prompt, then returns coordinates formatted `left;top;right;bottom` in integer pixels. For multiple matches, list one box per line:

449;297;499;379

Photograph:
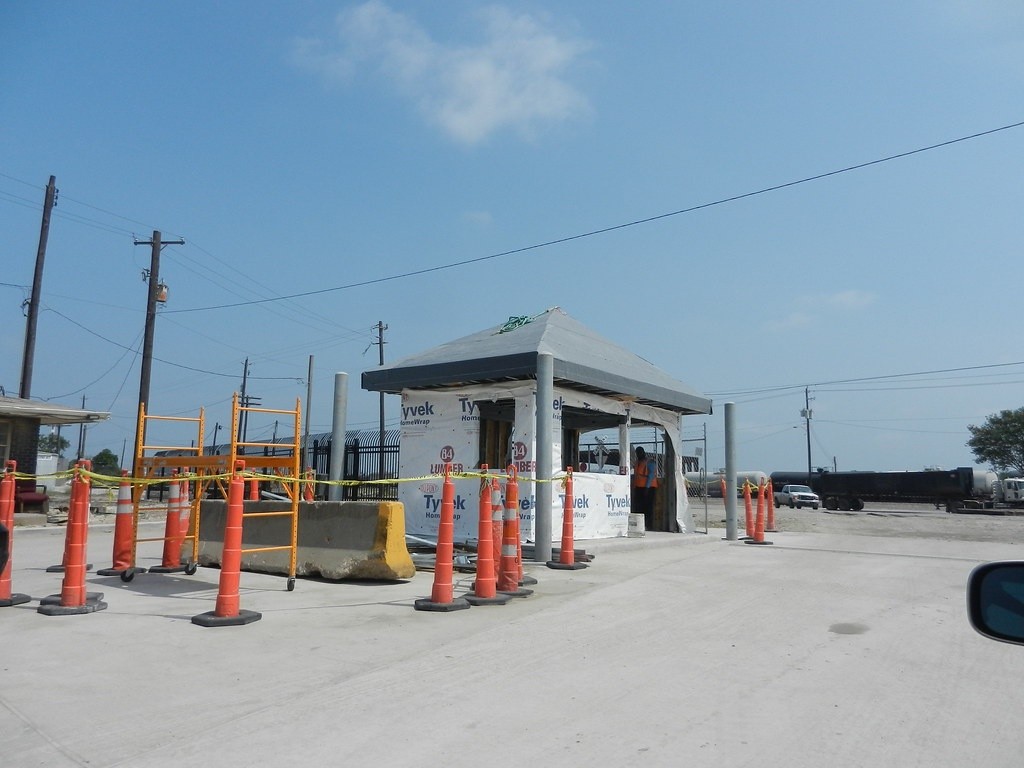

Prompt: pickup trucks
773;485;820;510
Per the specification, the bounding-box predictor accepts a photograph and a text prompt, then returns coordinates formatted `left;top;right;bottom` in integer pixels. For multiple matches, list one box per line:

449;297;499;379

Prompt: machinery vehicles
686;467;1024;516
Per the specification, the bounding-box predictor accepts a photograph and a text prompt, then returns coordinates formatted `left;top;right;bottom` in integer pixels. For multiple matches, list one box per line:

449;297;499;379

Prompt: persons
633;447;659;532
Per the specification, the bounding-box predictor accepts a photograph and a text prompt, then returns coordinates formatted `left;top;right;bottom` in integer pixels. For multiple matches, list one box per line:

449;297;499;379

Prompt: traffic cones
177;464;189;543
471;472;504;588
97;470;146;575
152;468;189;573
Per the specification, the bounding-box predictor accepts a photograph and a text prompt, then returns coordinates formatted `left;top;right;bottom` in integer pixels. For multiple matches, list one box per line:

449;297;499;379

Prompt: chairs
15;484;50;513
